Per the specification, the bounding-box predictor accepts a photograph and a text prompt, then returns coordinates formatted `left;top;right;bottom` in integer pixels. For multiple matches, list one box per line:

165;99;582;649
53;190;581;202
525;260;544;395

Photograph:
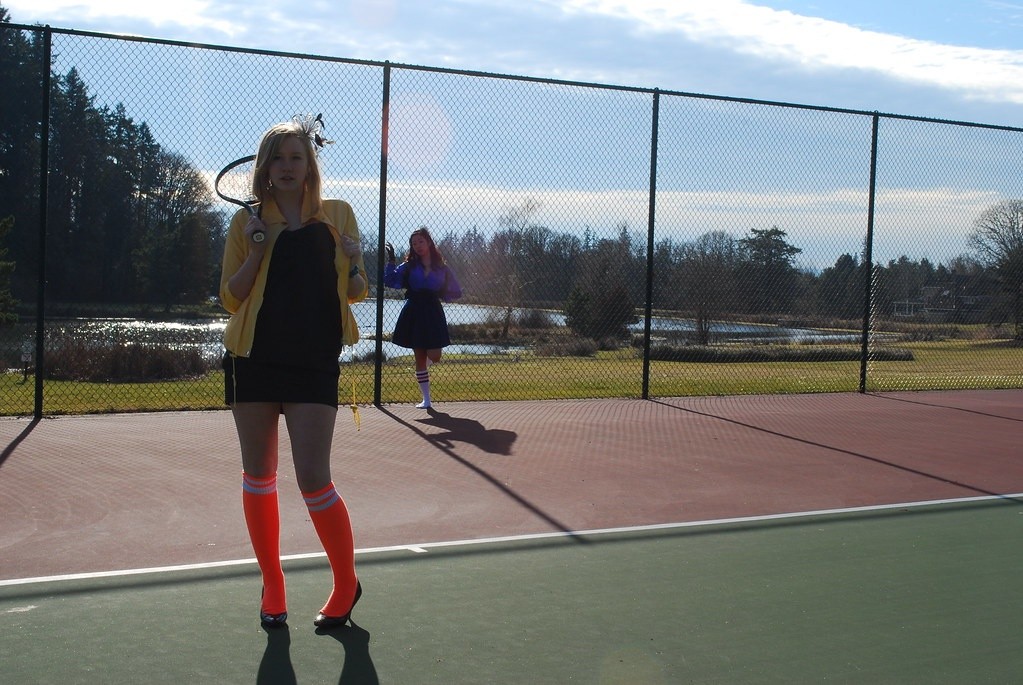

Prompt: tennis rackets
214;154;266;244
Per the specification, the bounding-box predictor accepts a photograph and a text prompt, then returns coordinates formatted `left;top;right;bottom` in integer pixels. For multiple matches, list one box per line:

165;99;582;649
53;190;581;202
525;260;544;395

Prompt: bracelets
349;265;359;278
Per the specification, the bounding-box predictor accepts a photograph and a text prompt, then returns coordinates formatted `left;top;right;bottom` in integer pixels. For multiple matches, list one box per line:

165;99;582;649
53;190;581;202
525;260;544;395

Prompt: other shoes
415;403;432;408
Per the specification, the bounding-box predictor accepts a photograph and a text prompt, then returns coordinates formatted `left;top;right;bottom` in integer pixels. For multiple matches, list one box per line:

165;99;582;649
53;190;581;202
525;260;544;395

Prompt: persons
219;122;369;628
385;228;463;408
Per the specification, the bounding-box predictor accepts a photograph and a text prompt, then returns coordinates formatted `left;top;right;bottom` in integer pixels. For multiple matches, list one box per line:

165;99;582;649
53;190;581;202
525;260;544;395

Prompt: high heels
260;585;287;625
314;578;362;628
